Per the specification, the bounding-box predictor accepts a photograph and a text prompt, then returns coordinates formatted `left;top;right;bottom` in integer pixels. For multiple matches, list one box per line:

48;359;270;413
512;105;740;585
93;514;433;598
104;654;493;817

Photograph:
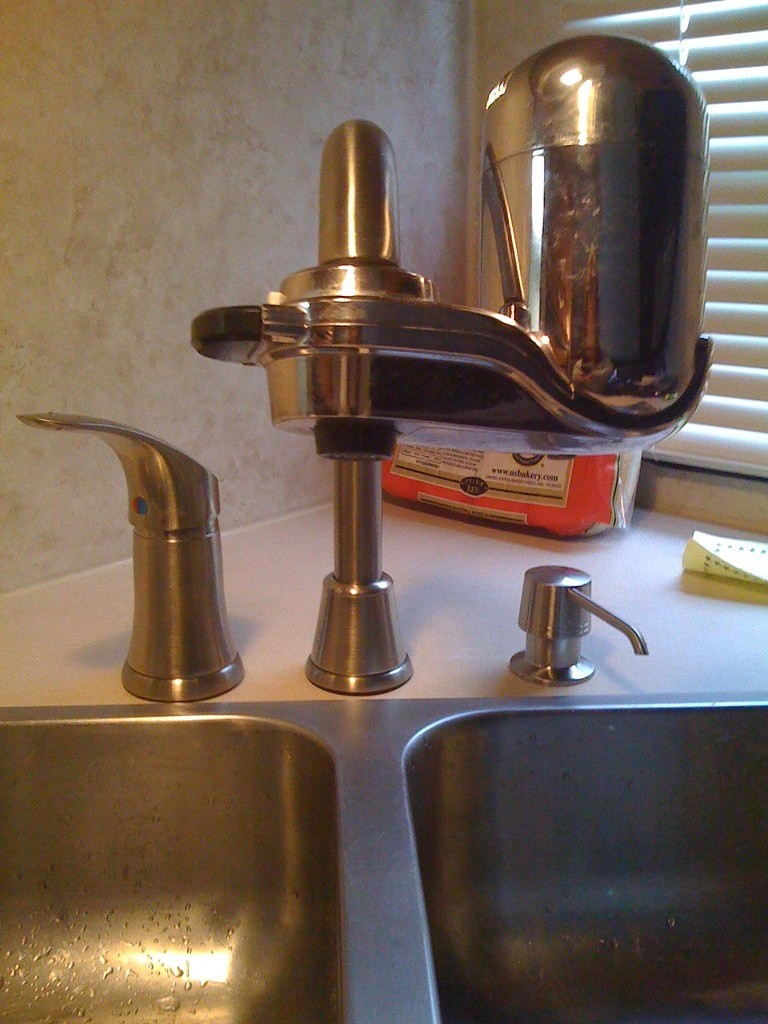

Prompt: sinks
372;694;768;1024
0;700;374;1024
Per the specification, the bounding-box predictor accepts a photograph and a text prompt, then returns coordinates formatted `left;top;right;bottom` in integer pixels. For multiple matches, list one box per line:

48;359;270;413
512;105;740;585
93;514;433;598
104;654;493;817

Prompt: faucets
191;120;713;695
